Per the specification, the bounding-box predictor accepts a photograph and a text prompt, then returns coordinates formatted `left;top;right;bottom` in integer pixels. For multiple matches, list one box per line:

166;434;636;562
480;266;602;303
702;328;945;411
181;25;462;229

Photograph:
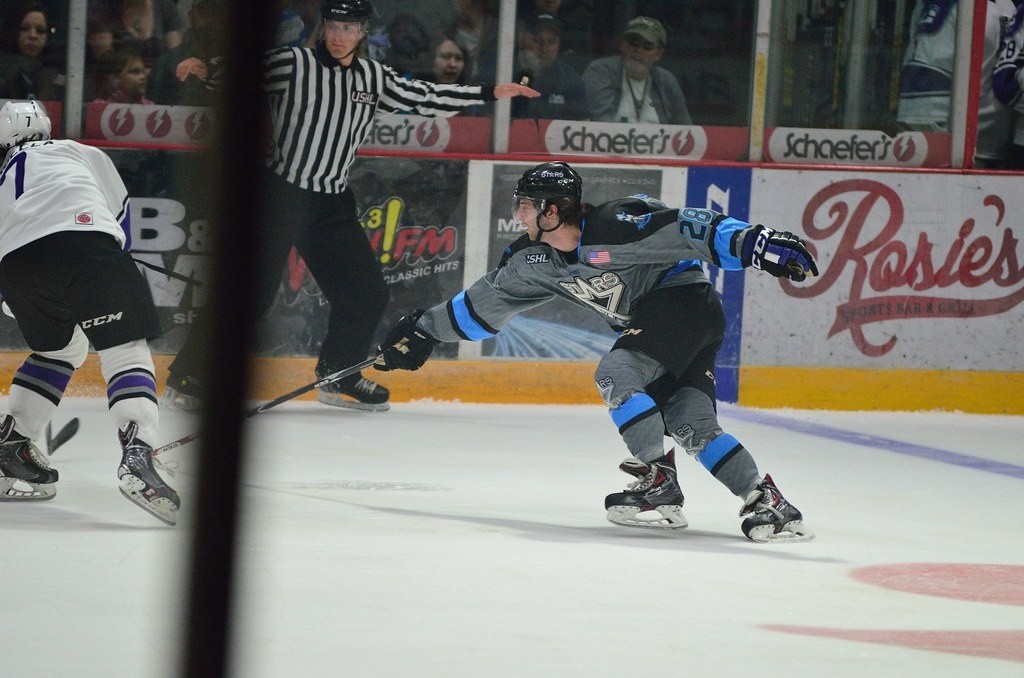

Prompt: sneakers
116;420;181;527
0;413;59;500
606;447;687;530
317;355;390;411
741;473;814;543
161;371;205;412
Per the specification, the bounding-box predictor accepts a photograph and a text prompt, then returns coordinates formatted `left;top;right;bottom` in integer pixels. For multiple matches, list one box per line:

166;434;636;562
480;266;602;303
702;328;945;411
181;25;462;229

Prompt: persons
888;0;1024;170
0;0;697;126
0;100;180;525
373;159;819;544
160;0;541;414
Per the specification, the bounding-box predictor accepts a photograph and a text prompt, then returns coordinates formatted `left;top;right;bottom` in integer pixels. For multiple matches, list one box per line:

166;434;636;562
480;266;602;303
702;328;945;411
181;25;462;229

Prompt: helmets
0;99;51;149
515;160;581;212
321;0;372;25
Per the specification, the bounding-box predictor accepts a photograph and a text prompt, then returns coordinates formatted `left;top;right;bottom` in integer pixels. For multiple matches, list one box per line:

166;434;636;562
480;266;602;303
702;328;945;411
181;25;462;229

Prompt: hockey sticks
152;350;385;457
45;417;80;456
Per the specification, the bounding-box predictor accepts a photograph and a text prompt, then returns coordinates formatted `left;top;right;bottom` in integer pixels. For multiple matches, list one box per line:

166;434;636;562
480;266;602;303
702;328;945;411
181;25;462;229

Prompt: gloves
373;309;440;370
742;224;820;281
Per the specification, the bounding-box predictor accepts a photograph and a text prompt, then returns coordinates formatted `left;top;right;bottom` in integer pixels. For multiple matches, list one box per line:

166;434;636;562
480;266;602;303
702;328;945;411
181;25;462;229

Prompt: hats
623;16;666;49
524;12;564;37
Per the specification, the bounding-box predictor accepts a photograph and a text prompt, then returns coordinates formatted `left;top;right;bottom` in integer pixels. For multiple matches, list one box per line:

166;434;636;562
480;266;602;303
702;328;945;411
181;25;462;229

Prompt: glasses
626;38;654;51
